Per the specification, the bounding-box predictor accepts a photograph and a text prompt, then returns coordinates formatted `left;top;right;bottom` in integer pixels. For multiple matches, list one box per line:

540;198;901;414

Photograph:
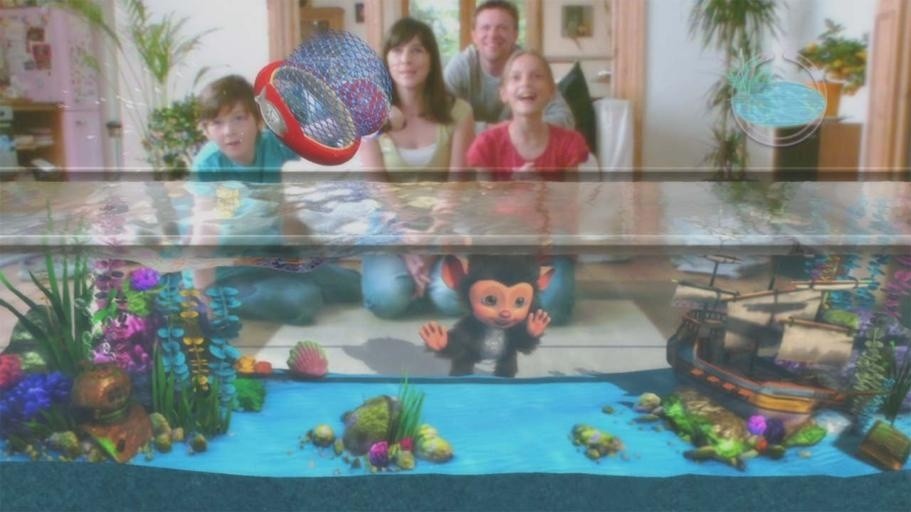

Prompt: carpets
251;298;674;377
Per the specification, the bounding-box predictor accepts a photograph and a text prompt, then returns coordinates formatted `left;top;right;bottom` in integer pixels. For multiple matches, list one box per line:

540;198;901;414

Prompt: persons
444;1;575;134
467;47;590;327
183;75;361;326
361;19;476;323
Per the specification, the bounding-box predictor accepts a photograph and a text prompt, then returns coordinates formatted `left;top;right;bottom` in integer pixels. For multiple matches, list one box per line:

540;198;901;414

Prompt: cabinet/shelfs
0;101;67;212
299;7;345;45
771;121;863;279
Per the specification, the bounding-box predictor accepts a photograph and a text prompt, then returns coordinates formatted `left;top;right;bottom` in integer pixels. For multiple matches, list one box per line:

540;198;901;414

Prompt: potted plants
662;0;791;280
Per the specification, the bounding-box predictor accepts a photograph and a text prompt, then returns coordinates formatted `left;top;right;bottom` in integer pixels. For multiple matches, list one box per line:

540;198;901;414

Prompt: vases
807;80;842;117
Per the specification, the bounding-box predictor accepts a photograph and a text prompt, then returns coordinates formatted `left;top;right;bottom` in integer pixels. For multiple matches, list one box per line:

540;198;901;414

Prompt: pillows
558;62;596;154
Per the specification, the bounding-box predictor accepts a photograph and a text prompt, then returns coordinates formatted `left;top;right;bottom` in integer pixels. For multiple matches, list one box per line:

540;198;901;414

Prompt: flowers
795;35;866;88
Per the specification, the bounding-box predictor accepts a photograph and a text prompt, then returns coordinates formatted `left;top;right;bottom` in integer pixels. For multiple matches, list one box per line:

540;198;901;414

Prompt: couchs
278;96;640;264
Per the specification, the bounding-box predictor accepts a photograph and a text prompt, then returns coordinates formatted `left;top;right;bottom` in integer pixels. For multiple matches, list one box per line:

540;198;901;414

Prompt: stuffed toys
419;254;552;378
